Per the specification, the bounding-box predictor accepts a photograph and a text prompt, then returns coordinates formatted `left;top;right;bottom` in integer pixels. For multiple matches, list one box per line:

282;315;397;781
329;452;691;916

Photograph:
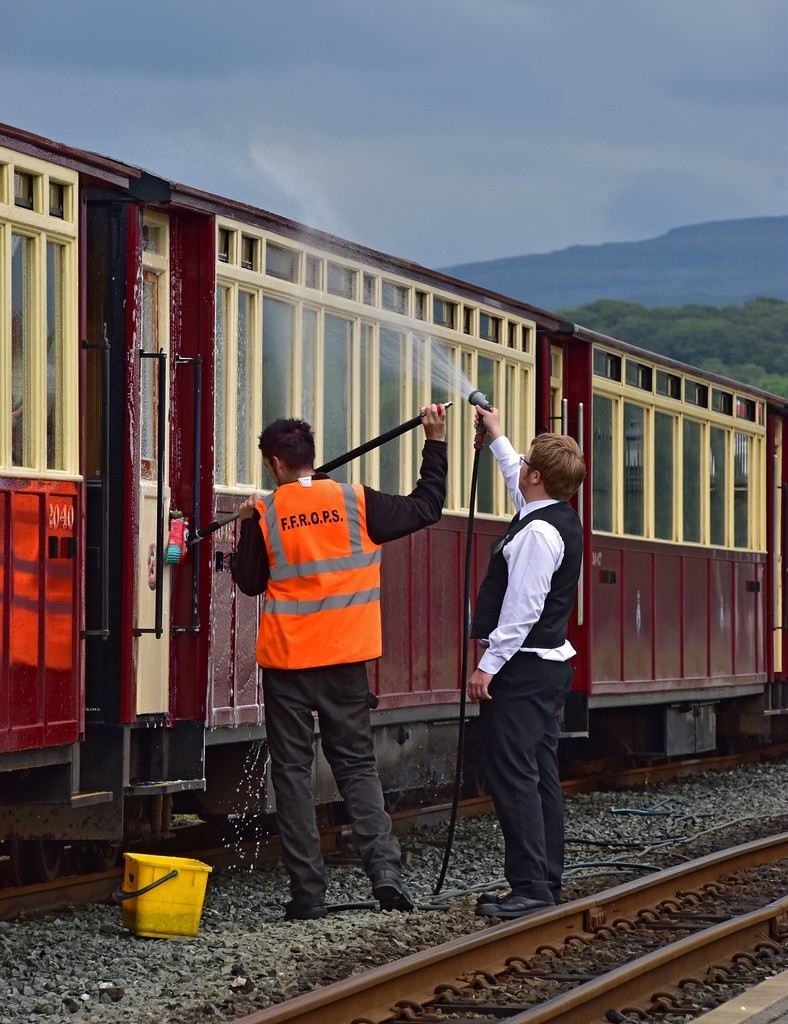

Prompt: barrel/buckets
115;853;213;941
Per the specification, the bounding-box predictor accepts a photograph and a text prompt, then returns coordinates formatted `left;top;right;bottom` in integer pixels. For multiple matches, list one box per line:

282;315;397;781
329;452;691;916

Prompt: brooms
163;400;455;563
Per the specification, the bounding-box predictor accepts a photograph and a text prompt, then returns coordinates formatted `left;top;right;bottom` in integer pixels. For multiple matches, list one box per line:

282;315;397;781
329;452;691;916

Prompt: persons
464;400;589;918
226;402;448;921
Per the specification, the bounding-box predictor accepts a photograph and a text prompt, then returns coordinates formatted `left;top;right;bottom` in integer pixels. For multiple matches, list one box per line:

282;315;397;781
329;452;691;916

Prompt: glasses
519;455;532;467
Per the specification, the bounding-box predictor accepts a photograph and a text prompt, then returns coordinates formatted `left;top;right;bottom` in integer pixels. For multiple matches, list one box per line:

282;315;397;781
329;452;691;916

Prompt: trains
0;120;788;892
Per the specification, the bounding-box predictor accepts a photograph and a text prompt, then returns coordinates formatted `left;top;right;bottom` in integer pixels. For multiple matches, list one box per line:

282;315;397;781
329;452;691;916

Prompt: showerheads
468;389;493;414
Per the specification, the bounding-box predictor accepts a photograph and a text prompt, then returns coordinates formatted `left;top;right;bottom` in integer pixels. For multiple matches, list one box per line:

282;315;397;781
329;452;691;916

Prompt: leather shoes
284;899;328;919
372;869;415;910
475;892;563;917
